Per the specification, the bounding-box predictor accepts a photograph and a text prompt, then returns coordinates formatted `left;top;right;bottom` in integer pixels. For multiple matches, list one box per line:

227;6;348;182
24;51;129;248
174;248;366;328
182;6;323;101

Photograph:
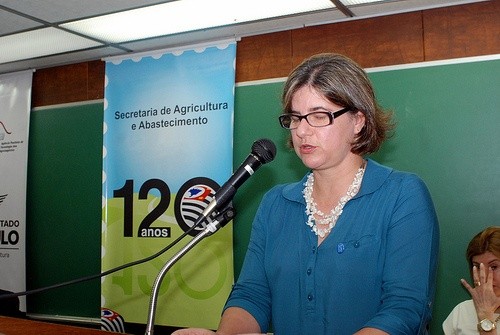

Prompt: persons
442;227;500;335
171;53;439;335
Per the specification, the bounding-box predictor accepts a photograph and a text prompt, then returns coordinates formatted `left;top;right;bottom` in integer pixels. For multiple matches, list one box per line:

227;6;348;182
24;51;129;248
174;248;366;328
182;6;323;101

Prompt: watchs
478;319;497;332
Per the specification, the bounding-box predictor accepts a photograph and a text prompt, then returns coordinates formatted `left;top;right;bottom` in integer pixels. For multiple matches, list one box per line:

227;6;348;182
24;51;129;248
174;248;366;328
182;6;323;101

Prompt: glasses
279;107;351;130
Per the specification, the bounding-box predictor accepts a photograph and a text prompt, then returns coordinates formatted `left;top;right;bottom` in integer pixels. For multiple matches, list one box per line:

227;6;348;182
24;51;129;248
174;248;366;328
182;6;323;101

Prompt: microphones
145;219;220;335
196;138;277;226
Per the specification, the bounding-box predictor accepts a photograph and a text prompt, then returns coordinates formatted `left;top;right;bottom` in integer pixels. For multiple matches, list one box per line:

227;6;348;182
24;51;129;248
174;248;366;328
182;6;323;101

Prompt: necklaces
302;158;365;239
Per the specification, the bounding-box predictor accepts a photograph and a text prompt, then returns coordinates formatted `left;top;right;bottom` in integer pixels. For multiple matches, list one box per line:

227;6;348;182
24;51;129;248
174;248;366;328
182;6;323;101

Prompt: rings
474;282;480;287
